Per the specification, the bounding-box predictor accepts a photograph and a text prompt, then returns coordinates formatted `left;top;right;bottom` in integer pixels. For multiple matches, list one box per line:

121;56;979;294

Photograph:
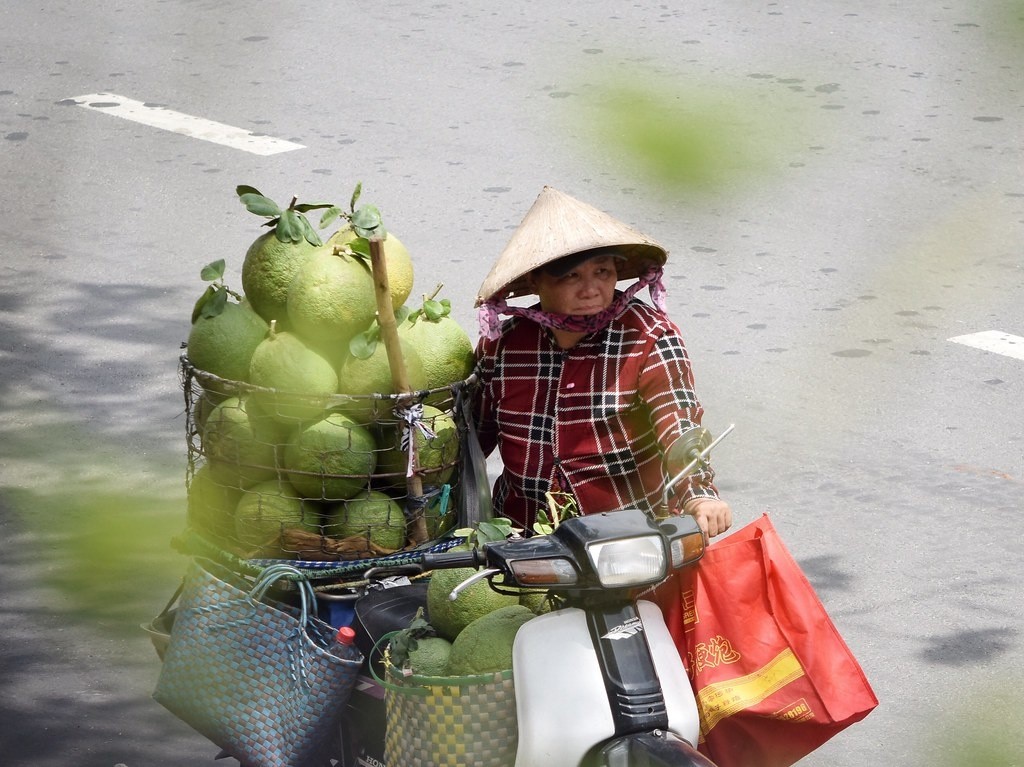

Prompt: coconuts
186;223;475;556
393;541;562;678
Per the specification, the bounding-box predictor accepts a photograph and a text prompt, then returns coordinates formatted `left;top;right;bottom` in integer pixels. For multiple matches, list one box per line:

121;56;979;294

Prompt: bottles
326;625;355;659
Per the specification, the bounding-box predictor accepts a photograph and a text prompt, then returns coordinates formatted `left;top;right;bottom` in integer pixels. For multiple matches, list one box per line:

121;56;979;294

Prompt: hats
476;183;667;307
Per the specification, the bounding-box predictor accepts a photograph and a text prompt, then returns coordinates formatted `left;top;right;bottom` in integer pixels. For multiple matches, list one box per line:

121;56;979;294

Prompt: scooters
214;418;735;767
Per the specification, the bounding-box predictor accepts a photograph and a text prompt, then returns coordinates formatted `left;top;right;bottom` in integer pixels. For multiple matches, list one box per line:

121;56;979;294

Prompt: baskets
369;627;518;767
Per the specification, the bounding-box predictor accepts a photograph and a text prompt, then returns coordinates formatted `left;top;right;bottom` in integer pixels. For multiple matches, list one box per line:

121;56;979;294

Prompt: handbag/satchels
626;511;880;767
149;553;364;766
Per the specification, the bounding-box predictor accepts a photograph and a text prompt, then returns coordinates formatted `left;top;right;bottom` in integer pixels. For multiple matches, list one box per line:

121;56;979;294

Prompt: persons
474;186;732;548
